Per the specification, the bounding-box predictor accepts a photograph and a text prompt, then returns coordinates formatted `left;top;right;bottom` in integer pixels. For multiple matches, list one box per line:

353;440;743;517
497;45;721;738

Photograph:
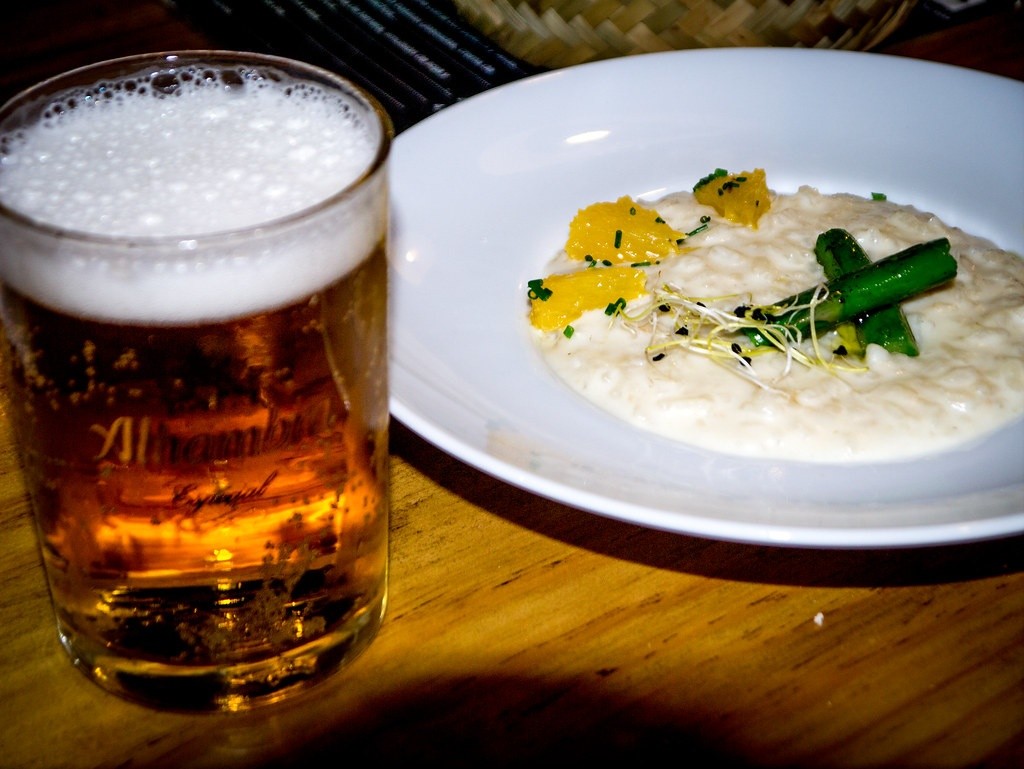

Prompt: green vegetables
742;227;960;359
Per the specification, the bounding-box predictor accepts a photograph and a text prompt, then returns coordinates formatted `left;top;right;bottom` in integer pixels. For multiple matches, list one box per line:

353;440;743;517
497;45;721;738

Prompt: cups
1;48;397;716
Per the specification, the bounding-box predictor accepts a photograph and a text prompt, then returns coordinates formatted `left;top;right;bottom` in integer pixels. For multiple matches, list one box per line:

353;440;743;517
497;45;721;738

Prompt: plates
386;45;1024;548
